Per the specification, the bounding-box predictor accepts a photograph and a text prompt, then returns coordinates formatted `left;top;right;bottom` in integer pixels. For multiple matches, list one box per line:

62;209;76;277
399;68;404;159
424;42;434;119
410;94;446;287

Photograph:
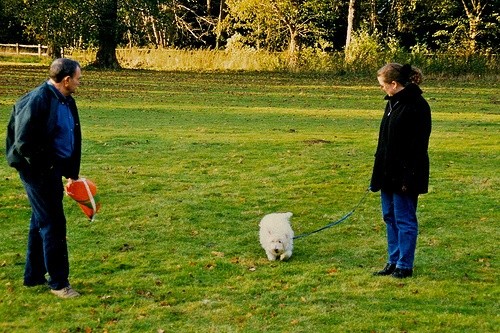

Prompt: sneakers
50;288;80;299
373;263;395;276
390;268;413;278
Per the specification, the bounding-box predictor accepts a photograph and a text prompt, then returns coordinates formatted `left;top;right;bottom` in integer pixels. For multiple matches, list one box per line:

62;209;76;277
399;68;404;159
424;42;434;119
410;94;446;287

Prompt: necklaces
387;99;398;116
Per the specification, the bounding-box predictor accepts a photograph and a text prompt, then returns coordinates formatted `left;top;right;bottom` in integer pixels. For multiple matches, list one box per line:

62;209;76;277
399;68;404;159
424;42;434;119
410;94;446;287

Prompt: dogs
258;211;295;261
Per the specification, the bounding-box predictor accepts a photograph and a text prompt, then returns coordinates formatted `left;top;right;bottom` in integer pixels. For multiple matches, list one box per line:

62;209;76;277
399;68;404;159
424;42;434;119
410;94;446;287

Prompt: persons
6;59;82;299
370;63;431;277
